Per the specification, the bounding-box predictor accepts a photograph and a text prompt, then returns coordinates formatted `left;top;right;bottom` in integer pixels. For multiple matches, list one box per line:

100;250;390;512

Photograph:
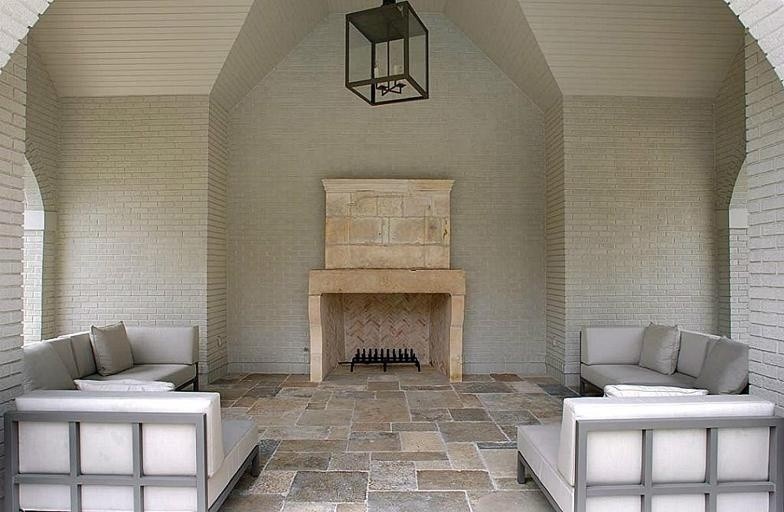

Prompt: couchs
6;389;258;512
22;324;200;393
579;325;750;397
516;394;784;512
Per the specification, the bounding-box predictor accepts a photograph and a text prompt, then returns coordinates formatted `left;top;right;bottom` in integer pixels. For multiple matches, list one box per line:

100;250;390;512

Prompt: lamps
345;0;429;107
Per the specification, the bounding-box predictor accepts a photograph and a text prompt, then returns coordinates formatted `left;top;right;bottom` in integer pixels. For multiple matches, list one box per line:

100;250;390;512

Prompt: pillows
603;384;708;397
21;340;75;392
693;334;750;394
73;378;174;393
90;322;134;376
639;322;679;375
